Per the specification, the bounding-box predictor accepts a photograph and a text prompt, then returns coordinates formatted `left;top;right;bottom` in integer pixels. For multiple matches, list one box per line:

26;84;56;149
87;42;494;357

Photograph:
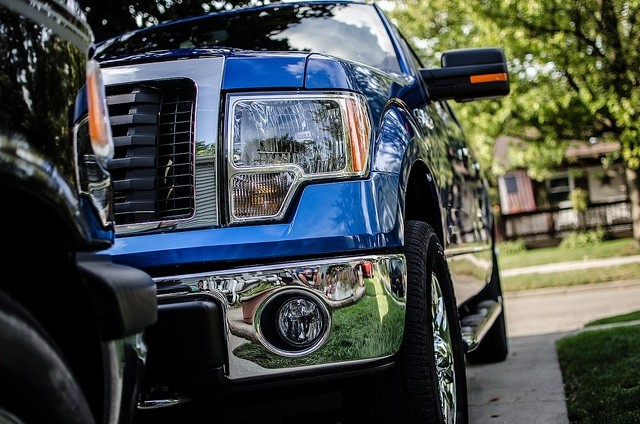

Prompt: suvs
94;0;508;424
0;0;156;423
